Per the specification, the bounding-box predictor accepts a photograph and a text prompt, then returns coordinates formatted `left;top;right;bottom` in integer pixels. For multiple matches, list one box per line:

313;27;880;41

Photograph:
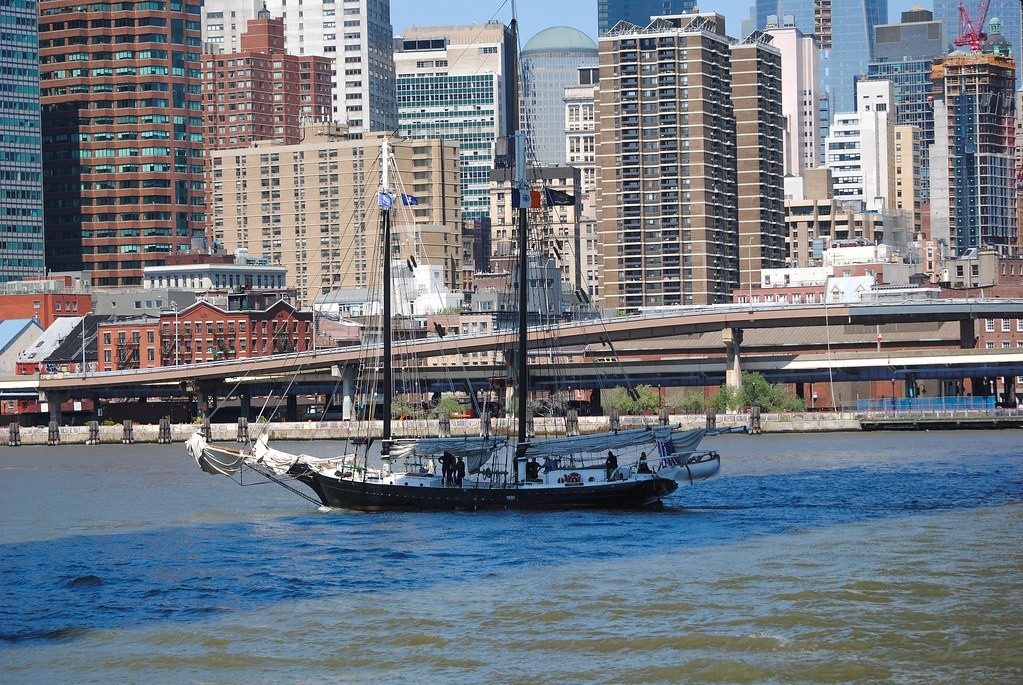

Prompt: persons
513;456;555;479
437;451;466;487
605;451;618;480
637;452;651;474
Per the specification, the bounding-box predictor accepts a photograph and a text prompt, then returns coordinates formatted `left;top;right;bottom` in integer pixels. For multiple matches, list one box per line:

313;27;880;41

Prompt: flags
656;437;683;466
545;188;576;207
378;192;395;207
401;193;418;206
511;188;542;208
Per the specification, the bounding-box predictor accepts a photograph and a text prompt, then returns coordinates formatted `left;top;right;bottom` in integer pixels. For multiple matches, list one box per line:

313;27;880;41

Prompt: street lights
82;312;93;374
158;296;178;369
749;238;754;304
313;287;324;350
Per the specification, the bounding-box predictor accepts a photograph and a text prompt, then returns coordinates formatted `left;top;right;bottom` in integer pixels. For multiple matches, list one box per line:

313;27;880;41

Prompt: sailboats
185;1;751;514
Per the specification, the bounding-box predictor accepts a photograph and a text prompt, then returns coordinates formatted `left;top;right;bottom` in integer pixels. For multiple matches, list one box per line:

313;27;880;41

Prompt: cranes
953;0;990;52
